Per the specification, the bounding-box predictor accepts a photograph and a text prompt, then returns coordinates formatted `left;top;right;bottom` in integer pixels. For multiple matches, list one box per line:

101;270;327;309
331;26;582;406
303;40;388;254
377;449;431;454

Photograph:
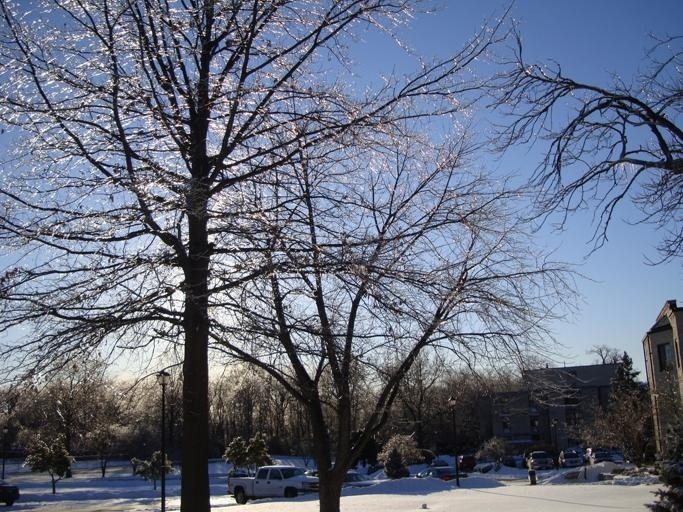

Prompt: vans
500;447;623;470
457;454;476;472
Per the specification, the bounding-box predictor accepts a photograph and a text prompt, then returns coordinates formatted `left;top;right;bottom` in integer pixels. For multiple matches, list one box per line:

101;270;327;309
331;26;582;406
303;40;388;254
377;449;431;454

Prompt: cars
0;478;20;506
414;466;468;481
304;462;375;487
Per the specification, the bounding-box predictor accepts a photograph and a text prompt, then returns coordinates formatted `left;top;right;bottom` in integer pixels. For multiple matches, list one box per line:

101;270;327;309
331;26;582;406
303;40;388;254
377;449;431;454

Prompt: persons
526;455;539;485
458;453;464;474
524;457;538;483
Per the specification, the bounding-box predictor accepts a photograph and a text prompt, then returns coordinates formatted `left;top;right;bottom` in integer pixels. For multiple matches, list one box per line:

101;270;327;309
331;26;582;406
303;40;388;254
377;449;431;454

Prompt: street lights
446;395;460;487
157;368;171;511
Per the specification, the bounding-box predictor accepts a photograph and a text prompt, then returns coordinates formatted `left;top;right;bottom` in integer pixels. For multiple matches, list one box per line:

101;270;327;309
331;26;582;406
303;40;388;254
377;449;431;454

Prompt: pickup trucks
227;464;320;505
